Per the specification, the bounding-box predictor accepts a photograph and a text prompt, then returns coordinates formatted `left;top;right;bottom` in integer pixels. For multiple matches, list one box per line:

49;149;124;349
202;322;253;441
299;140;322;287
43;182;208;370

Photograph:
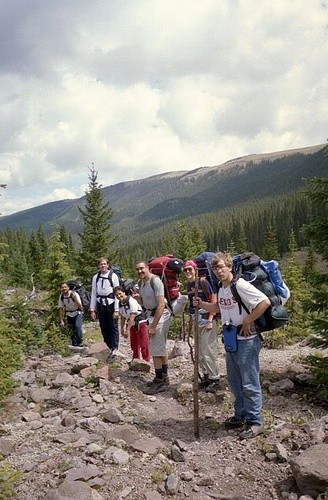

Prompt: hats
61;280;91;313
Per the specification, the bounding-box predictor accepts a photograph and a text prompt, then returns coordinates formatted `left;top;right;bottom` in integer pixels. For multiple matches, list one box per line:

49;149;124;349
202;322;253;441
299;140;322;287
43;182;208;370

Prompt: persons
130;261;171;395
193;253;271;438
114;286;150;363
89;257;120;363
58;282;85;347
182;261;222;392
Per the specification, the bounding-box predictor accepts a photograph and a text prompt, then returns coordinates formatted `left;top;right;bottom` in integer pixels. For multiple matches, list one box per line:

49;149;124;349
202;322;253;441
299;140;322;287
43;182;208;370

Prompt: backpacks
119;278;147;320
139;254;189;316
186;252;220;319
216;251;291;335
96;264;123;299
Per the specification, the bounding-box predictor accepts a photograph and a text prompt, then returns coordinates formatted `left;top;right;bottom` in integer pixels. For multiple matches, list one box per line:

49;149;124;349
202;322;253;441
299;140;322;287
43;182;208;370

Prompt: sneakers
137;372;170;394
238;422;264;439
223;416;242;429
198;374;222;393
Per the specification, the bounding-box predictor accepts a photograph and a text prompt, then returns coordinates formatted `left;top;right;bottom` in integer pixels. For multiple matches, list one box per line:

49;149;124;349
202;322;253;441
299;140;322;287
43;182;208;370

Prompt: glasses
183;267;193;272
212;264;225;270
